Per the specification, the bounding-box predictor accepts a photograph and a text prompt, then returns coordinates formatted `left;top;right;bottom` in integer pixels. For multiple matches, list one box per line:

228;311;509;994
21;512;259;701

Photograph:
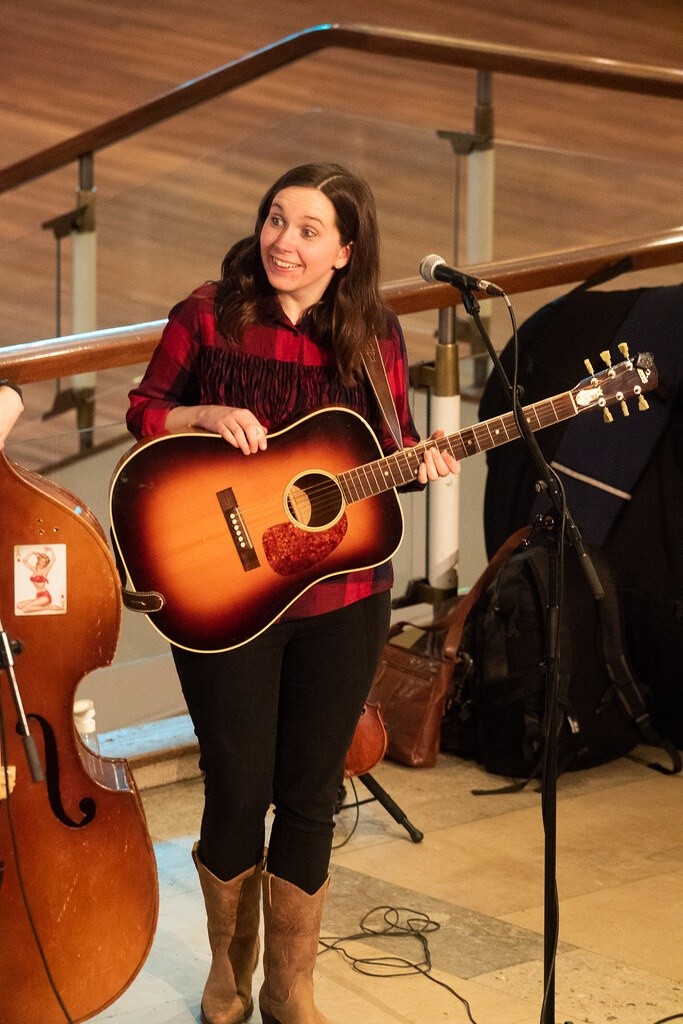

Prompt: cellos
0;372;160;1024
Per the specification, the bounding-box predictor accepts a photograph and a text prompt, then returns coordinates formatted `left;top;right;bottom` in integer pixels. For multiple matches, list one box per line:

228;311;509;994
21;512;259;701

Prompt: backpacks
449;547;681;787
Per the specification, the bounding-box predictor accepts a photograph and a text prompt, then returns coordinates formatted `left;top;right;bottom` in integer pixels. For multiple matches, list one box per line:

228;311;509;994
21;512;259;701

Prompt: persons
126;161;461;1023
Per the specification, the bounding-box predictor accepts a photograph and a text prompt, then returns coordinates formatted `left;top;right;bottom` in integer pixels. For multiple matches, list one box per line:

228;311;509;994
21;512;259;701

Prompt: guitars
107;337;661;658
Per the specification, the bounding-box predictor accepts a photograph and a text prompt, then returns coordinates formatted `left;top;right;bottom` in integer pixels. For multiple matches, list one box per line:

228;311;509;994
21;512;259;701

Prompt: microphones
419;253;504;298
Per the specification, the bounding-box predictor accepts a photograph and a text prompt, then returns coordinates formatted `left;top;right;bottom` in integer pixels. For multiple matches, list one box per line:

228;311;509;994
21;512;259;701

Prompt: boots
258;868;331;1024
192;839;269;1024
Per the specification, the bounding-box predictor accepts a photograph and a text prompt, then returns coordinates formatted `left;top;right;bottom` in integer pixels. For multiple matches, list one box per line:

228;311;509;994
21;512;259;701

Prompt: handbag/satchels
366;526;537;768
